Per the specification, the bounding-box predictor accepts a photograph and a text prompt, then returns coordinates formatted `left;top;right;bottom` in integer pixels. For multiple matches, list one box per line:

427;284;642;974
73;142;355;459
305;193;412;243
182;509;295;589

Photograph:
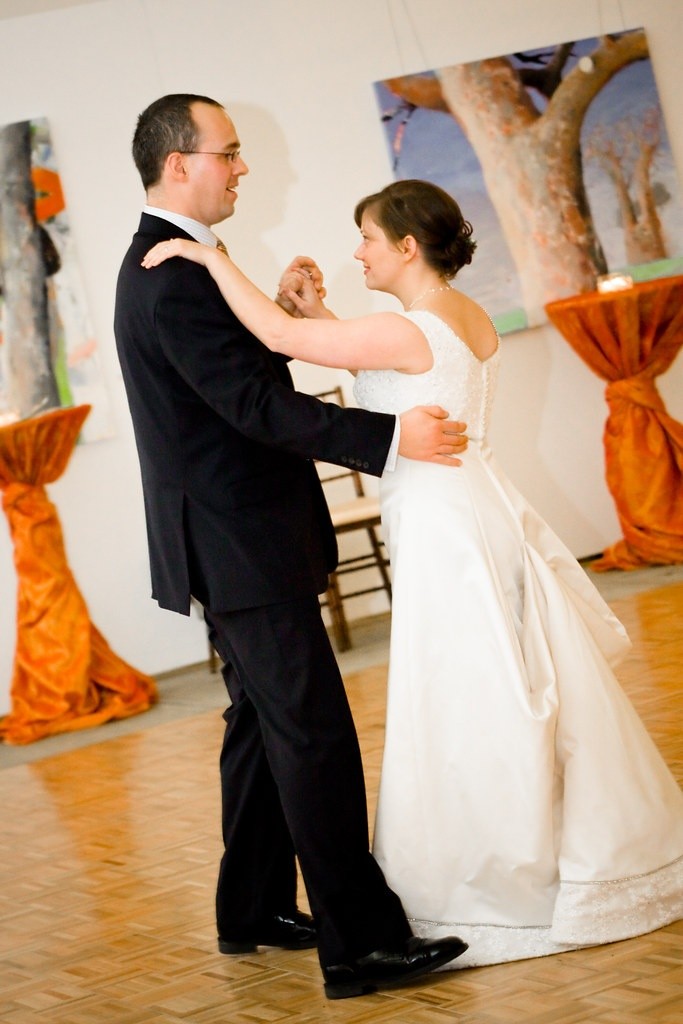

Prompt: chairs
315;385;392;653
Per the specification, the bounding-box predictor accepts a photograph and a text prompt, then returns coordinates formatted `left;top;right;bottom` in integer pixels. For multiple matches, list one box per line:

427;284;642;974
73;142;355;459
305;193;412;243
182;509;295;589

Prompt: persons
142;179;683;970
116;95;468;1000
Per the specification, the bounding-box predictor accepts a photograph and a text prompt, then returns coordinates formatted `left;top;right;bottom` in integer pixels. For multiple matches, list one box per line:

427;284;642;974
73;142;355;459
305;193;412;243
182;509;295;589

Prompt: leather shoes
214;905;320;954
322;934;470;999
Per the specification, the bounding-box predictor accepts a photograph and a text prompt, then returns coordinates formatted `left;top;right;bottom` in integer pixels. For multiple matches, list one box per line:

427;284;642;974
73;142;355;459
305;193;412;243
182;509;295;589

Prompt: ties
216;240;228;257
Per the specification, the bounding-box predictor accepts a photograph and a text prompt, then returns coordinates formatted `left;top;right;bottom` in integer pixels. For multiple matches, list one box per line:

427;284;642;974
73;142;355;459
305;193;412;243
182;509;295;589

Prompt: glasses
174;150;241;163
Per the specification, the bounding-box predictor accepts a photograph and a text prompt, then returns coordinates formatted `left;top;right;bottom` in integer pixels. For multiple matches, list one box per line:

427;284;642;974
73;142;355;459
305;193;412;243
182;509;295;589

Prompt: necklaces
408;286;455;309
